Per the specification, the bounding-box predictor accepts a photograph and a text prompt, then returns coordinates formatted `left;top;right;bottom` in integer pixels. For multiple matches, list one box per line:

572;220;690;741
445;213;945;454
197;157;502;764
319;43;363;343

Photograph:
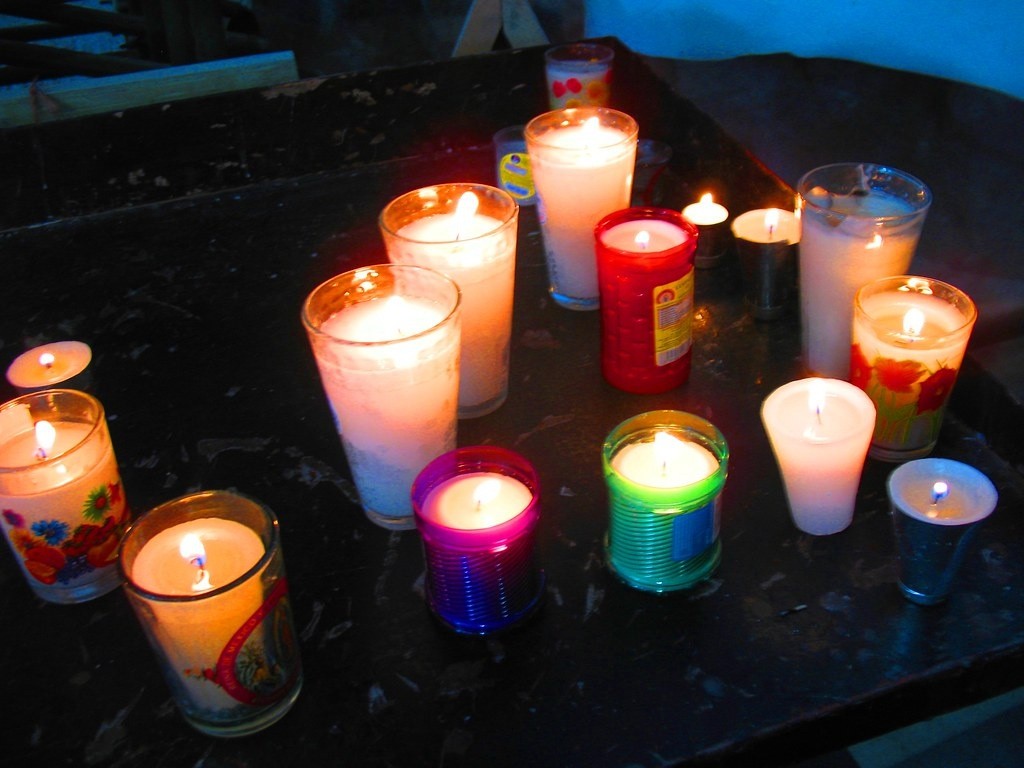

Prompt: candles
0;42;999;739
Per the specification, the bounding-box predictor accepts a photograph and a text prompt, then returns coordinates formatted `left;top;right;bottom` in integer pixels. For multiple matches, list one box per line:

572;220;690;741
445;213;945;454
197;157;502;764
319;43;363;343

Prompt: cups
524;107;639;311
116;491;304;736
379;183;518;418
602;410;729;592
796;162;932;378
493;125;539;205
412;446;540;635
887;458;998;605
761;376;877;535
731;207;799;321
851;276;977;461
593;207;698;394
681;202;729;269
545;42;614;110
6;341;109;418
300;264;460;530
0;389;133;602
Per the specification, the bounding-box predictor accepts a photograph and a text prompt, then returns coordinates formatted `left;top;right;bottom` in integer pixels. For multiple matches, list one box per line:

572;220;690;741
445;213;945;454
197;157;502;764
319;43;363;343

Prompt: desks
0;36;1024;767
